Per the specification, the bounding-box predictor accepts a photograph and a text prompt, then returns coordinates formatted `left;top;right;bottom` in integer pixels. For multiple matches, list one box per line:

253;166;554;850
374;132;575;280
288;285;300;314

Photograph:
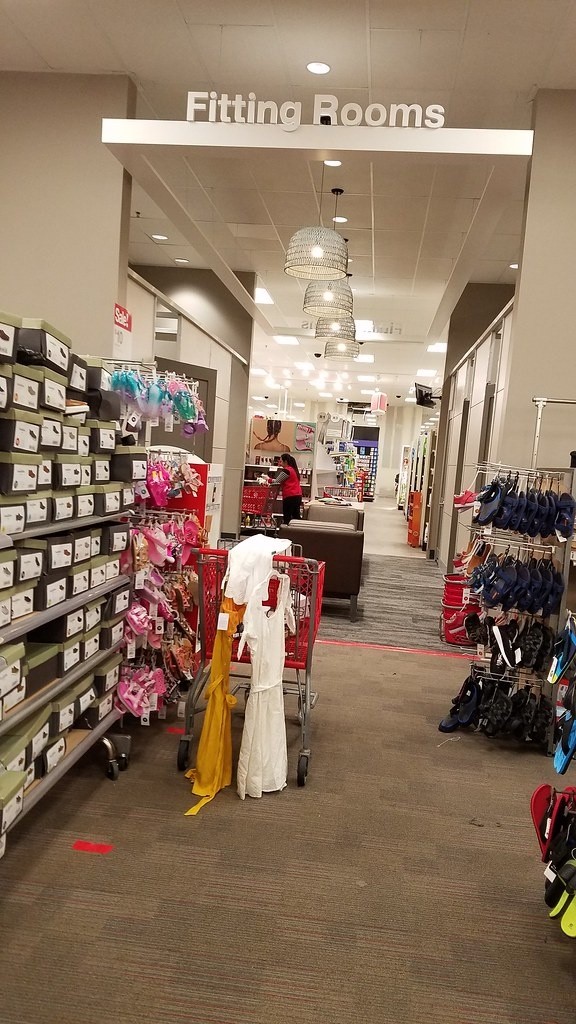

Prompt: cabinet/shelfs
0;511;131;859
396;431;438;552
241;464;314;531
343;475;364;503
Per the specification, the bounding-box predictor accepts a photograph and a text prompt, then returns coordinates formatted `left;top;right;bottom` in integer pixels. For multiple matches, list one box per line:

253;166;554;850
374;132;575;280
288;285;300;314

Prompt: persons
261;453;303;526
253;420;290;452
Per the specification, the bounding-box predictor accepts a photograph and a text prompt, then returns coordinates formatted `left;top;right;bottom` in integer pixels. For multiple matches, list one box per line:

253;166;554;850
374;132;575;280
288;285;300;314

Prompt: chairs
279;505;364;623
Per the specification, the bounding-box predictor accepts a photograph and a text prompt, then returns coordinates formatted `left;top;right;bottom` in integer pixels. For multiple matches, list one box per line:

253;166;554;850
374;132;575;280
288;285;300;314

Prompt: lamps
283;160;359;361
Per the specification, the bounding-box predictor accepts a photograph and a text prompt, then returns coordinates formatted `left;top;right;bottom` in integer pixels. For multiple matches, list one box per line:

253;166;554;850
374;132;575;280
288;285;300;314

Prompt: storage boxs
0;310;146;837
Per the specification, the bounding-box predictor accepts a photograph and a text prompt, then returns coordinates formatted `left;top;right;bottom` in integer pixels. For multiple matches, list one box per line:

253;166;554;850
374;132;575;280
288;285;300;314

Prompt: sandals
437;469;576;938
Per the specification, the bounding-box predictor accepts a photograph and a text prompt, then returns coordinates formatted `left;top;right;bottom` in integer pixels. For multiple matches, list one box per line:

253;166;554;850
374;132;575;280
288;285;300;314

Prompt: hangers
260;553;283;584
220;551;230;590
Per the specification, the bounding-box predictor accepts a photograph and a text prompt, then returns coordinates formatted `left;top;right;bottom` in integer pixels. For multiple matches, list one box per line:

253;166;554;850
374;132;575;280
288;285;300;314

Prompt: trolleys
184;542;327;790
242;483;280;527
323;485;361;502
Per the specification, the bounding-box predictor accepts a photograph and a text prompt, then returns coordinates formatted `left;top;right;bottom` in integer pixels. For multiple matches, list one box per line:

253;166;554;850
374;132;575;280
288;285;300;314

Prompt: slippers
111;360;210;725
